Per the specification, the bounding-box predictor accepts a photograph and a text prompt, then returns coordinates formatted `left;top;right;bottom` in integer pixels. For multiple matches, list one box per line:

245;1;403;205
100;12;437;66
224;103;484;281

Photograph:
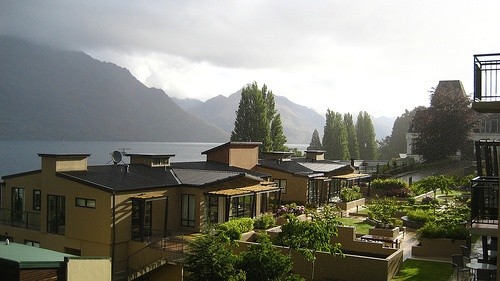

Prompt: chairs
460;245;478;265
450;254;471;281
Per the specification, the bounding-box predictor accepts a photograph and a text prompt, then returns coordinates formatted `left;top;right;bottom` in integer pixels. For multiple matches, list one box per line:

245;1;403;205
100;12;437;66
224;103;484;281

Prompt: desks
361;234;396;249
466;262;498;281
